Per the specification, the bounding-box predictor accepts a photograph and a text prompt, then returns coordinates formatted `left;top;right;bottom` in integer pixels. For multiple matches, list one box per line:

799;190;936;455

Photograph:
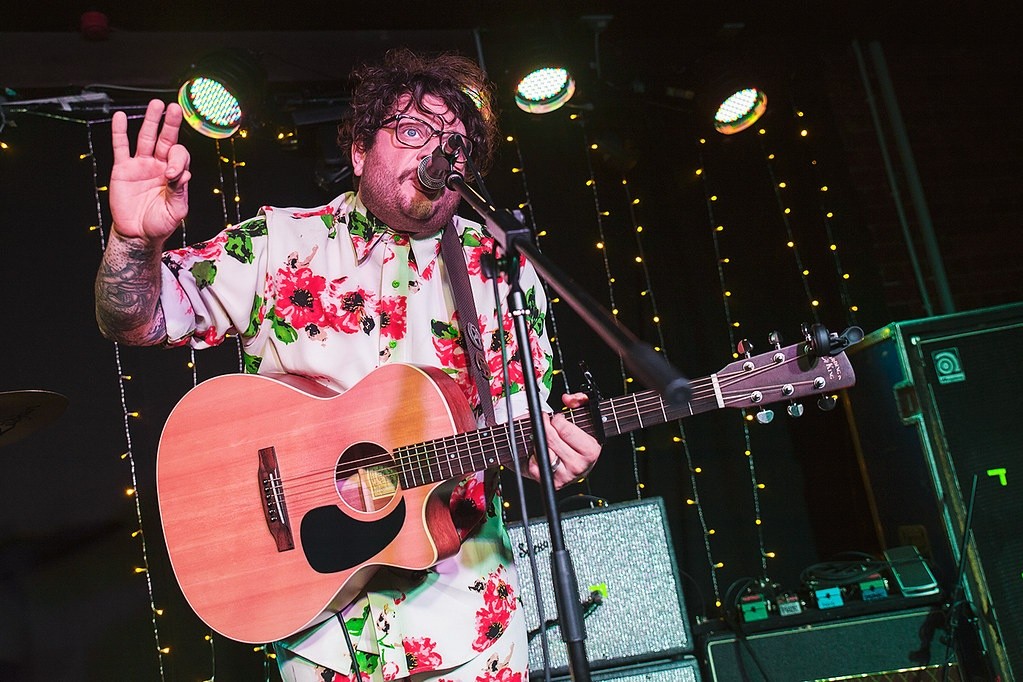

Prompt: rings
551;456;561;472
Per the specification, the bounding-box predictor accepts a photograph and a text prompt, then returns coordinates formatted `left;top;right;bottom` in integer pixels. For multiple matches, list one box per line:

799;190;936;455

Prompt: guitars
154;320;868;649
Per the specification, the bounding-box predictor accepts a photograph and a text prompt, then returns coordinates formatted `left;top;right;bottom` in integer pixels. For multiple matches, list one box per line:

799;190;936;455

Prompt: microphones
591;591;603;607
417;133;462;190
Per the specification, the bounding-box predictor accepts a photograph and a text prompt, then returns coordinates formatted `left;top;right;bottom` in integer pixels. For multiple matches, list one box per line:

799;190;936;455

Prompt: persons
93;50;600;682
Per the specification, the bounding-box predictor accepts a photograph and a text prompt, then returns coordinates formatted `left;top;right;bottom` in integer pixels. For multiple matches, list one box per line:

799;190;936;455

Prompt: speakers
498;296;1023;682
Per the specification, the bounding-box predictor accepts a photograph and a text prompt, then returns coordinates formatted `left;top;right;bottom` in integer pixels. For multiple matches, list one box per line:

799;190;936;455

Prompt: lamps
175;47;267;140
699;67;766;134
508;43;576;114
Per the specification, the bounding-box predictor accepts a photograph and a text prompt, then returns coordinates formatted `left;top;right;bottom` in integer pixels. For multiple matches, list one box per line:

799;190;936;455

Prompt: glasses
375;114;473;164
